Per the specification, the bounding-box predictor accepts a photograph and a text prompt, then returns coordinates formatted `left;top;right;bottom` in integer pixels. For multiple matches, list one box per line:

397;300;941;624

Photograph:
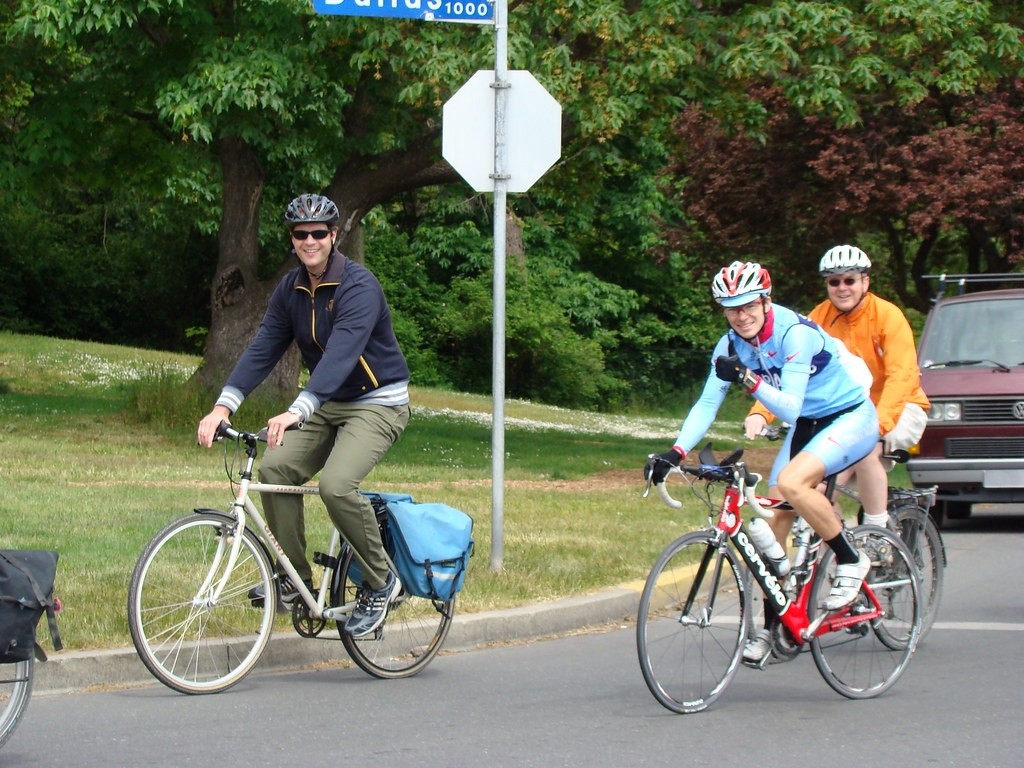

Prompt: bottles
749;515;789;576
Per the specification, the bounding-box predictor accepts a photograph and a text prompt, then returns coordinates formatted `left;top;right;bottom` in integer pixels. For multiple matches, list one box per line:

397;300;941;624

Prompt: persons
741;242;929;564
198;192;410;638
643;261;879;664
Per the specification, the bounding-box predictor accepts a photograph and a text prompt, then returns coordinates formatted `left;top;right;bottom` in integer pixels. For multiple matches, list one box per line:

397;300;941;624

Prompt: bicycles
635;440;923;716
125;419;458;696
745;423;948;652
0;548;37;752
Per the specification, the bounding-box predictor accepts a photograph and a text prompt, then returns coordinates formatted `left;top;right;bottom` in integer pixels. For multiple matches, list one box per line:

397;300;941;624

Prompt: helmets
819;244;871;275
282;193;340;229
710;260;772;307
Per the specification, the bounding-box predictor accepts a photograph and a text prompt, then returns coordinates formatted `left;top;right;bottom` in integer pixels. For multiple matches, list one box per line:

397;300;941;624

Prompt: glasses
722;298;764;316
290;229;331;240
826;275;866;287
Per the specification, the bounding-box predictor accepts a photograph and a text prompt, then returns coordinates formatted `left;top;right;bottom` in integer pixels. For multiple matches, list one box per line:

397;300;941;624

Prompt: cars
905;269;1024;528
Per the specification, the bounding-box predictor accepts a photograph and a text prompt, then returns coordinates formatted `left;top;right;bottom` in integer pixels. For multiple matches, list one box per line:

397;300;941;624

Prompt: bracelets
742;370;761;392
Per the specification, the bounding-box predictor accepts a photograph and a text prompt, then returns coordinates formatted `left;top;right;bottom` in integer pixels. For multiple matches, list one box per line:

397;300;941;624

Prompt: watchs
287;406;303;419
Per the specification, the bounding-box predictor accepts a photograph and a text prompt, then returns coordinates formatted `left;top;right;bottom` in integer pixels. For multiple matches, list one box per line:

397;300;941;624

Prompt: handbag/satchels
0;548;64;664
383;499;476;620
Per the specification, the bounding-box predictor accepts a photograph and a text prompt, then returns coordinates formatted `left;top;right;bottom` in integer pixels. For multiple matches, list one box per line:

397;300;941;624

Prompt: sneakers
741;630;782;664
344;570;402;637
821;549;872;610
245;569;315;603
855;533;894;568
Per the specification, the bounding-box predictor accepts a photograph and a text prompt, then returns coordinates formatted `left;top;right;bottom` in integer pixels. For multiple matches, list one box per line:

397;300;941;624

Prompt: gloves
644;449;684;486
714;338;747;383
